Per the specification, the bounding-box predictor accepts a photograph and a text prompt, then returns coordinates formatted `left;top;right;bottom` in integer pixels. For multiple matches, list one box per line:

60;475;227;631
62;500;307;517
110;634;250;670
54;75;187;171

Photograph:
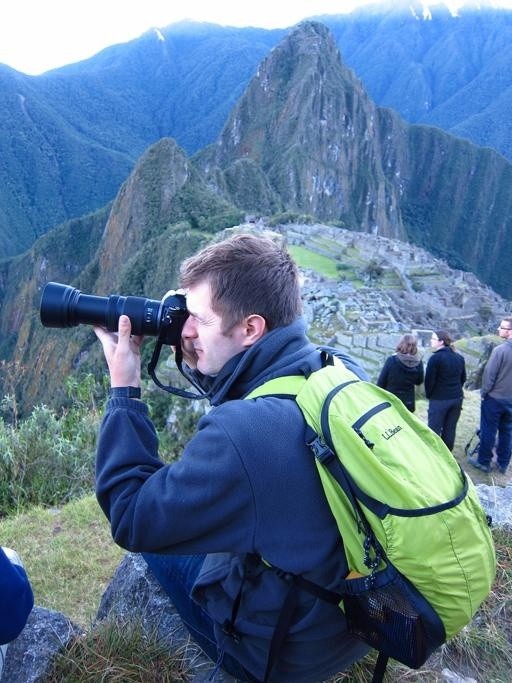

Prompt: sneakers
470;458;506;473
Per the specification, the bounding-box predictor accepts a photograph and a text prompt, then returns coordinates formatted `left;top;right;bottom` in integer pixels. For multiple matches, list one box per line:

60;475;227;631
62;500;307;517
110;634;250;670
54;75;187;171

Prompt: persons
423;330;467;454
467;315;512;474
375;332;425;412
90;232;375;683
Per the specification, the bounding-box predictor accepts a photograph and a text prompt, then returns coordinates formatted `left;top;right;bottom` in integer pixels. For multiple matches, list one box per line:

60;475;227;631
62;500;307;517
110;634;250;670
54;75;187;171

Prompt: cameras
40;282;187;348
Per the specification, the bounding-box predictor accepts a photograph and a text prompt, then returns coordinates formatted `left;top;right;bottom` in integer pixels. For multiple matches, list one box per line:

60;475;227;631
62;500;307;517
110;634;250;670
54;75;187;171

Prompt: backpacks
243;348;496;670
